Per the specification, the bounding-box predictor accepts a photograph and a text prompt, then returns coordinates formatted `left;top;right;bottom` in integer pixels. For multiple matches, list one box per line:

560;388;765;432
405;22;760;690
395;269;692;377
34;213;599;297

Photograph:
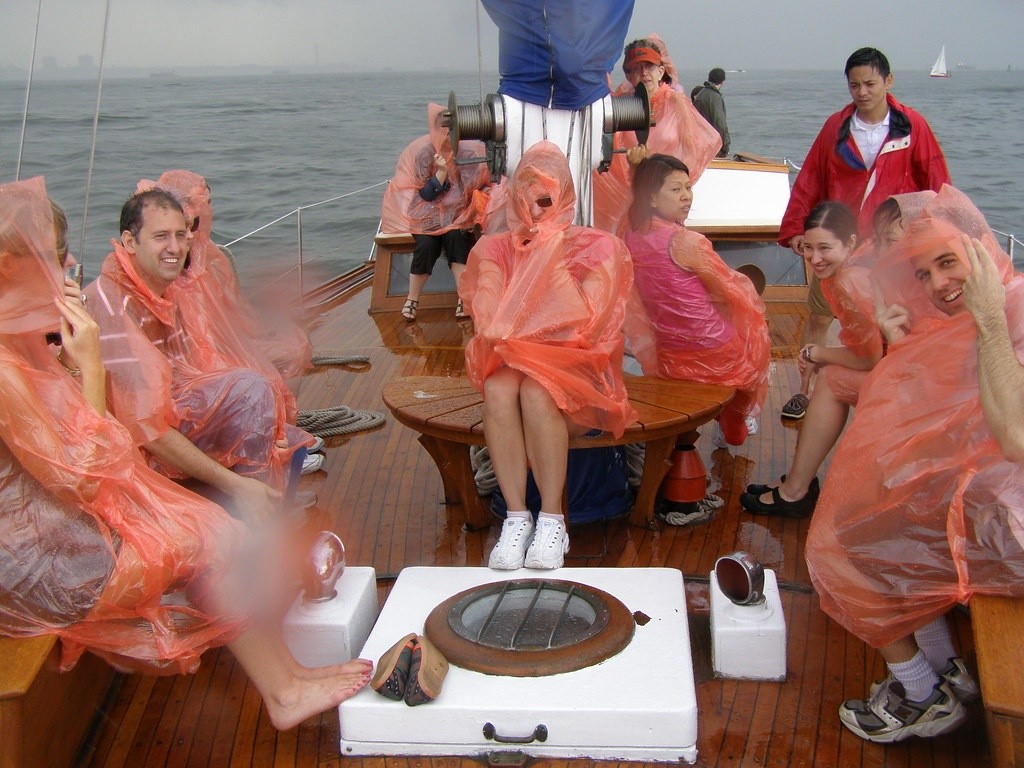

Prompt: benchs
969;596;1024;768
0;634;114;768
382;376;739;527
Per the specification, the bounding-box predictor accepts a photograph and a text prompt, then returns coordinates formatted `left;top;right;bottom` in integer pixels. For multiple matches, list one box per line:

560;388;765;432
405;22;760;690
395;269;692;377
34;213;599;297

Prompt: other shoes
307;436;324;453
370;633;450;706
300;454;325;474
739;473;820;517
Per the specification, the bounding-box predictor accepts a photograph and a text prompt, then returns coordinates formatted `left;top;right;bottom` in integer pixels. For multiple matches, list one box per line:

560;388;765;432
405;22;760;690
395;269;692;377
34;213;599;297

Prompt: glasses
8;243;68;267
631;63;657;73
535;198;552;207
191;216;199;232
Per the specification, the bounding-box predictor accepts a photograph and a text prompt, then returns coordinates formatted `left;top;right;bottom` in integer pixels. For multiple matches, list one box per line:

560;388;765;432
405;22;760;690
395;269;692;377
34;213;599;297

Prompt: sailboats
929;45;949;77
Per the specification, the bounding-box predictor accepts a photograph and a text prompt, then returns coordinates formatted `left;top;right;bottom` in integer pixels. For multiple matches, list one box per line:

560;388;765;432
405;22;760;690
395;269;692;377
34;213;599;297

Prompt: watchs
803;345;820;365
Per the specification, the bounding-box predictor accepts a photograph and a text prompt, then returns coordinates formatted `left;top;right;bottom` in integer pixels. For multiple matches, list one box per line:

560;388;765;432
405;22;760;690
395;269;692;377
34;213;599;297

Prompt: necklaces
649;88;657;116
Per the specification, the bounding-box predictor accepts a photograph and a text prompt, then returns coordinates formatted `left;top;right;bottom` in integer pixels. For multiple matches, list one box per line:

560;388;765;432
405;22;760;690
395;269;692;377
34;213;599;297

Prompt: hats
625;48;661;67
709;69;725;82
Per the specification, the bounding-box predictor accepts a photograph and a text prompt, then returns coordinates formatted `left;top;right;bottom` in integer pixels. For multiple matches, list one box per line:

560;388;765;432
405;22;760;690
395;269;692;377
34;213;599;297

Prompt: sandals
401;298;419;321
455;297;470;320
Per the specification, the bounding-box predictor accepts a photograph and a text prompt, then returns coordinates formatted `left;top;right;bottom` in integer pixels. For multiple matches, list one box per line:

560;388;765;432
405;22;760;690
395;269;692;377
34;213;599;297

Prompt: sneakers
781;394;809;417
839;675;966;742
869;658;978;705
489;516;534;569
525;517;570;570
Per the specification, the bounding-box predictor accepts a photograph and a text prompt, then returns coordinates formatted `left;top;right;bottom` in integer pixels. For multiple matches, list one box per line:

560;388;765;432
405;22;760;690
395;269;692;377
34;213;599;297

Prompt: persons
738;185;1024;744
627;153;770;451
738;47;951;419
602;33;731;235
0;169;373;730
402;107;489;323
473;141;616;572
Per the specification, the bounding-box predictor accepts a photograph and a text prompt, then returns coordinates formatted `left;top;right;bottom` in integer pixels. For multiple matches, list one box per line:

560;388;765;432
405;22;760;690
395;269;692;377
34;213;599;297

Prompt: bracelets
57;351;80;376
